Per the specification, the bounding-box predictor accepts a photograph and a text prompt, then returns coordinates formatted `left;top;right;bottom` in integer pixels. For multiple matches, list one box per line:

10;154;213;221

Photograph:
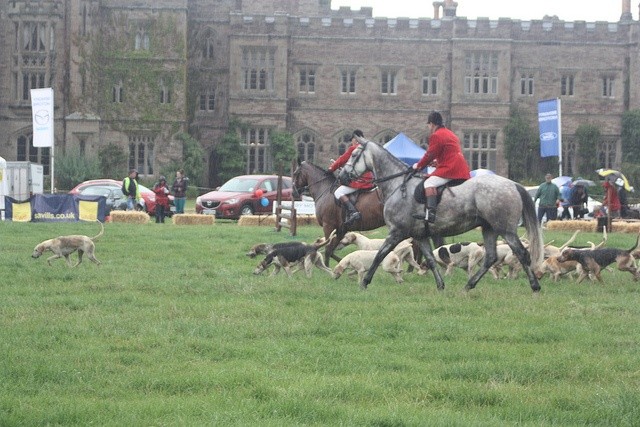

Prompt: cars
522;186;609;225
67;178;177;217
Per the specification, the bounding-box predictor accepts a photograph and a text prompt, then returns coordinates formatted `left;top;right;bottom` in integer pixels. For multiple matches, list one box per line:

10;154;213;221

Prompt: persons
413;111;470;222
172;169;188;213
600;182;620;218
571;187;589;219
153;176;170;223
122;169;139;211
561;181;573;220
620;186;630;219
328;130;374;225
533;173;559;226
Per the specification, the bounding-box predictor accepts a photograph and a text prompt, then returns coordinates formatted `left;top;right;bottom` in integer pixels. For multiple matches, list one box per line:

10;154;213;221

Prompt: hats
348;129;363;142
426;112;443;126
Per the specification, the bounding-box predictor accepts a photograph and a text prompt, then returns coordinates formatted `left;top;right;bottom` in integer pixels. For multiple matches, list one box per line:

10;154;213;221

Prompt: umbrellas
572;179;596;187
595;168;634;192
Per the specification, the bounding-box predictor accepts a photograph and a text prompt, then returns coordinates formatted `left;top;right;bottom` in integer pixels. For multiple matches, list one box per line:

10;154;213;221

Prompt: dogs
31;218;107;270
332;243;413;288
253;228;337;282
335;230;428;275
430;226;640;286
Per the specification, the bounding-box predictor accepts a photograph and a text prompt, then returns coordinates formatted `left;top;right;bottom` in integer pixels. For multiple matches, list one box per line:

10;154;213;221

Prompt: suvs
196;175;302;219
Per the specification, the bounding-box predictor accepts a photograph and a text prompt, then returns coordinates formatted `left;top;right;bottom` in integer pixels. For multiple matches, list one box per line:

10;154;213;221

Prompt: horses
336;133;548;297
290;156;425;274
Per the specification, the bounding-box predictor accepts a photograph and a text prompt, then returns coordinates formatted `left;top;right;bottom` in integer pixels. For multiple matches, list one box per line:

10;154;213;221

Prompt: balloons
255;190;263;198
260;196;269;206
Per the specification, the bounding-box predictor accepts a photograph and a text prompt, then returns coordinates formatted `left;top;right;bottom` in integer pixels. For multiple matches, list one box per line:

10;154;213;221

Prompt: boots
344;200;360;225
412;196;437;223
